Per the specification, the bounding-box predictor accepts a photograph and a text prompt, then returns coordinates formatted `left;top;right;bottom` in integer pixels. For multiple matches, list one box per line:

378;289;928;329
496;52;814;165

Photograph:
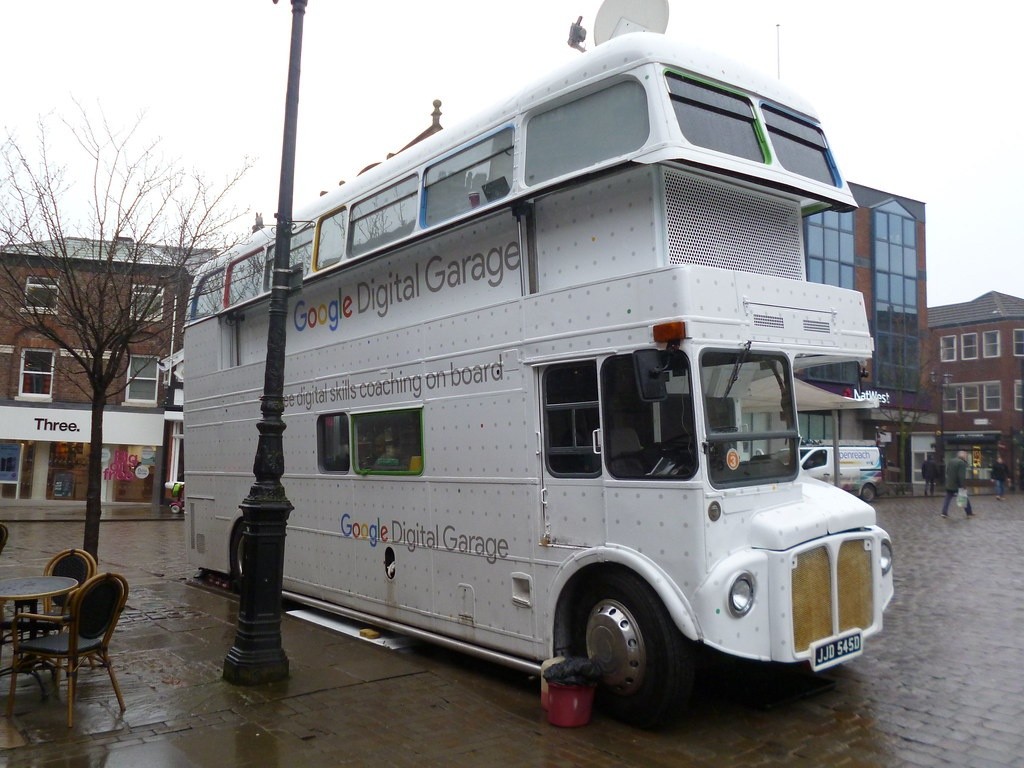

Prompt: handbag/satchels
957;488;967;508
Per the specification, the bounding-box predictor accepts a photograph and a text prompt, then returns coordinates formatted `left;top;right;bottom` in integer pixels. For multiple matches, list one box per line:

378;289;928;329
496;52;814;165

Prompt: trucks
183;0;893;730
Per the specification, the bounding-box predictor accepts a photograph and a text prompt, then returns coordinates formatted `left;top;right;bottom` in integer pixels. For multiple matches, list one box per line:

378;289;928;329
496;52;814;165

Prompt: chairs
606;408;643;477
7;548;129;727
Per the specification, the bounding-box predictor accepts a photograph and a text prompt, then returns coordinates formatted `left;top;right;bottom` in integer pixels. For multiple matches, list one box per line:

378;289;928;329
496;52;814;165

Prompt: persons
922;455;937;494
942;450;977;519
991;457;1011;500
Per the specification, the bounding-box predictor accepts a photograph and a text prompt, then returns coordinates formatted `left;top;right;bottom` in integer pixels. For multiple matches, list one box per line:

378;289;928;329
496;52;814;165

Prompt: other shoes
996;494;1008;501
966;514;976;519
941;513;948;518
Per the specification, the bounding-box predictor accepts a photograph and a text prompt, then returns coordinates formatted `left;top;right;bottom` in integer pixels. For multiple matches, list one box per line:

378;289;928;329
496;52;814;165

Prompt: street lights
930;370;954;487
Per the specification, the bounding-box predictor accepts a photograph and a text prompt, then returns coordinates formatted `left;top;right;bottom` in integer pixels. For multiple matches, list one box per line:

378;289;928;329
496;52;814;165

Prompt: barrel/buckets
547;683;594;726
541;656;567;710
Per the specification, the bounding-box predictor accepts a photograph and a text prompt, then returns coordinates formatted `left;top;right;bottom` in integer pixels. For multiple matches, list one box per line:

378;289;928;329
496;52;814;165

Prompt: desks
0;576;79;698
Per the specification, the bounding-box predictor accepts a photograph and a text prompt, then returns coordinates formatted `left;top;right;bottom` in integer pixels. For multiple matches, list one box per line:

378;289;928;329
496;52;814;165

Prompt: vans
775;446;885;503
